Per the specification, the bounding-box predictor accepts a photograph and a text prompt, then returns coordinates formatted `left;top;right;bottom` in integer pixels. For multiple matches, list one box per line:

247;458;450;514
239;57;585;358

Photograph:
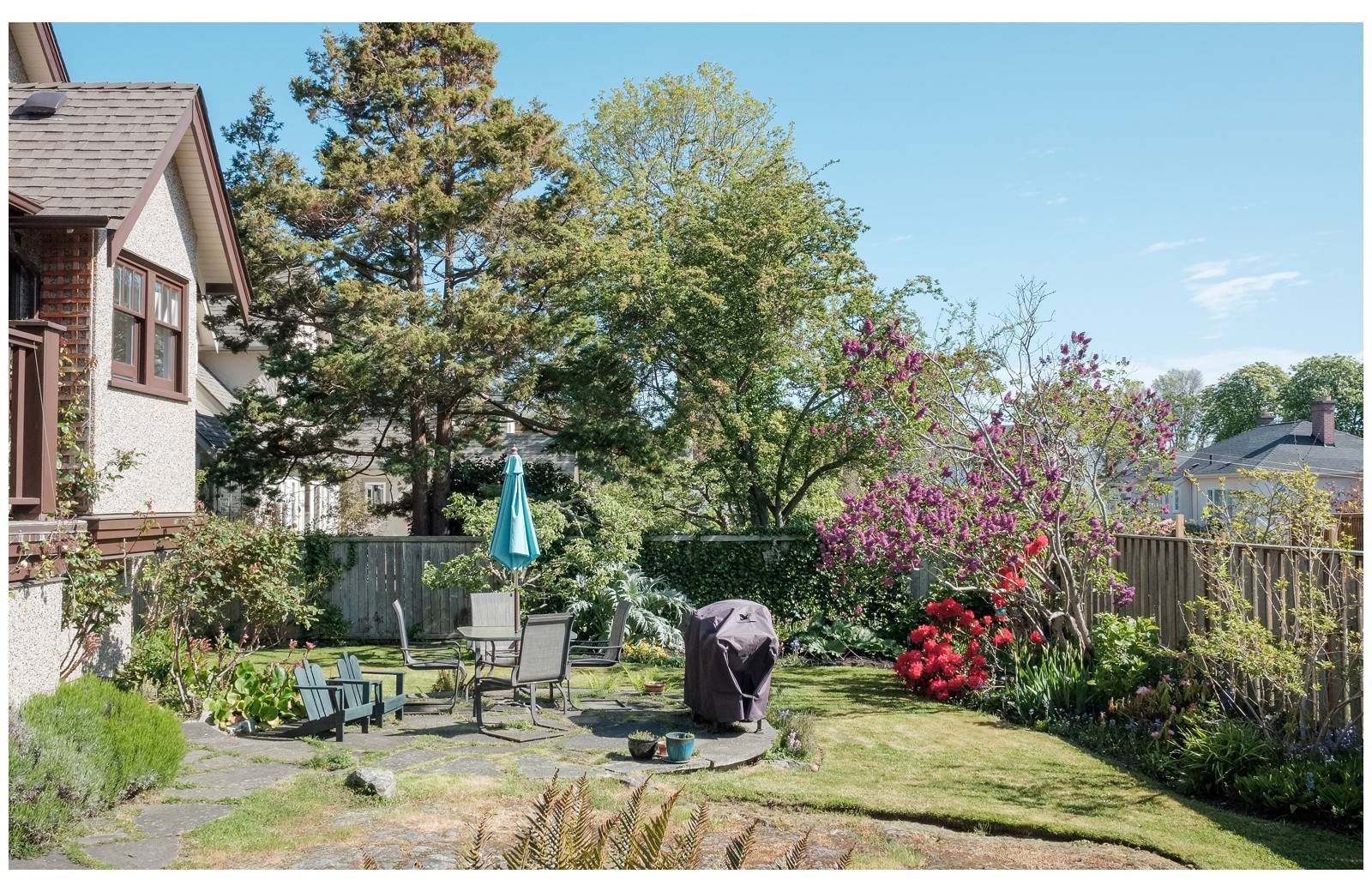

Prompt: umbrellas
489;444;541;666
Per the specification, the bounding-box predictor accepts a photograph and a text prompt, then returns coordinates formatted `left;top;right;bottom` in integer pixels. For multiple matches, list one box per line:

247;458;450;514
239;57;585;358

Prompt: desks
456;626;578;712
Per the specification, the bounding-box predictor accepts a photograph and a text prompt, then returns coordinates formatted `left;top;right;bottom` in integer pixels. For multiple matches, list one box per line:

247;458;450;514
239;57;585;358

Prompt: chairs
465;591;632;740
392;599;465;714
278;659;373;742
329;652;407;728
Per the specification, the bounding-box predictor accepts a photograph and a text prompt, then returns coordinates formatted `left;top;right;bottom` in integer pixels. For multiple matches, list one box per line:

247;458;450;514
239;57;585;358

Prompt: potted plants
657;737;667;757
628;729;655;761
666;731;695;761
644;678;670;692
